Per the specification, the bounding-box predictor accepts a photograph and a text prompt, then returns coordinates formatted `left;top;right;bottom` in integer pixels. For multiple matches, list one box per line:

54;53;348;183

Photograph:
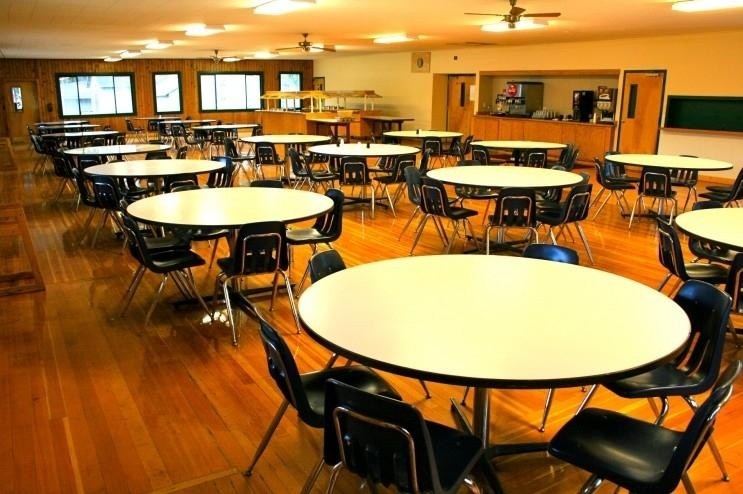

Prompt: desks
297;254;692;492
675;207;743;334
126;187;334;322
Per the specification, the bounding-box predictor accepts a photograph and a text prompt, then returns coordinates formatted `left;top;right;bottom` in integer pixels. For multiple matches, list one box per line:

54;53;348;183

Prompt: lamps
103;0;336;64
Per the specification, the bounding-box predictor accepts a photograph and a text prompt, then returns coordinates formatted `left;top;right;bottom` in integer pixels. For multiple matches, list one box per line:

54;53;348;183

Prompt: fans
464;0;561;29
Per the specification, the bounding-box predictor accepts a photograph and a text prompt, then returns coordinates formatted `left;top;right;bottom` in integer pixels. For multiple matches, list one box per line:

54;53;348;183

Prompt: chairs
272;189;345;297
110;212;195;309
718;252;742;348
120;214;214;333
211;220;301;346
654;217;731;298
576;280;731;482
308;249;432;400
242;318;404;493
547;356;742;494
322;379;484;493
27;116;743;265
460;243;588;433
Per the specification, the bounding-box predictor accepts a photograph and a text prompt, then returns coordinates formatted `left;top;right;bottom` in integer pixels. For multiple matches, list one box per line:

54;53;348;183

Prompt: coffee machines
572;90;593;122
